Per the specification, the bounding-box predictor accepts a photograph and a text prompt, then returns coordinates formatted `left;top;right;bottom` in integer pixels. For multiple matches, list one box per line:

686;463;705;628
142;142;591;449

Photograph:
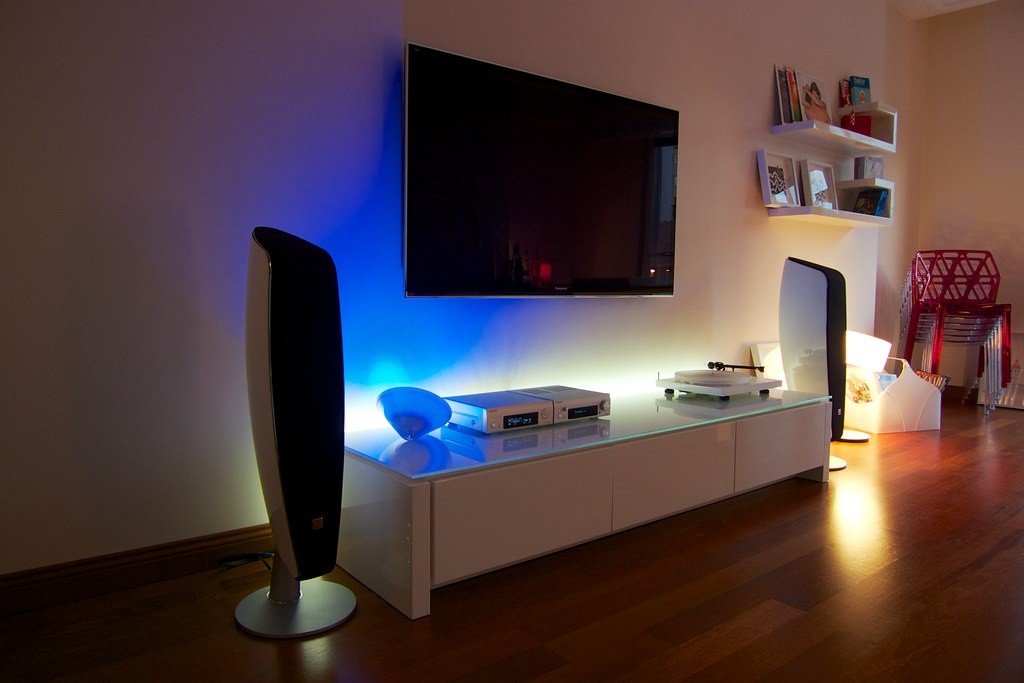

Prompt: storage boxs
840;115;872;136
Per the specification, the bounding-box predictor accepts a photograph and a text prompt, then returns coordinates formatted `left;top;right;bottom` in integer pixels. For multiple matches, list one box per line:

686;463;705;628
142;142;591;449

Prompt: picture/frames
800;158;838;210
853;189;888;216
756;148;801;208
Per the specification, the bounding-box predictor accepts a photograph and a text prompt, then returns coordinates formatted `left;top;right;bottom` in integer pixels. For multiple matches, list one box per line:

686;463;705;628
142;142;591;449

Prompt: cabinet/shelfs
337;387;833;620
767;101;898;227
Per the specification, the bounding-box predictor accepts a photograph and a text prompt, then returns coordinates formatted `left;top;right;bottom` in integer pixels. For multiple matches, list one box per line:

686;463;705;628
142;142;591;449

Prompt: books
850;75;871;106
854;156;884;180
774;63;832;126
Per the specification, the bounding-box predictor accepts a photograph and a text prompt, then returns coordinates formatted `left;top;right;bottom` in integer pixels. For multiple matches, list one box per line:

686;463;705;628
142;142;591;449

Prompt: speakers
234;225;357;640
778;256;847;471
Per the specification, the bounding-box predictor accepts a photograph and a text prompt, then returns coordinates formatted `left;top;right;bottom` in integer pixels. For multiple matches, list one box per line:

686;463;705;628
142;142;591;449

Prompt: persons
801;81;831;124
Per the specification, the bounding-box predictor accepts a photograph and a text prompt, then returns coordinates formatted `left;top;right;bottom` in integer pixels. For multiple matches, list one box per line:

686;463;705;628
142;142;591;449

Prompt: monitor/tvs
403;41;680;299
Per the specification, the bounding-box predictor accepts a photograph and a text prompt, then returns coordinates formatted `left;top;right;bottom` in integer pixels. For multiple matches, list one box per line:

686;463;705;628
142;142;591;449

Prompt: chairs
893;250;1012;415
